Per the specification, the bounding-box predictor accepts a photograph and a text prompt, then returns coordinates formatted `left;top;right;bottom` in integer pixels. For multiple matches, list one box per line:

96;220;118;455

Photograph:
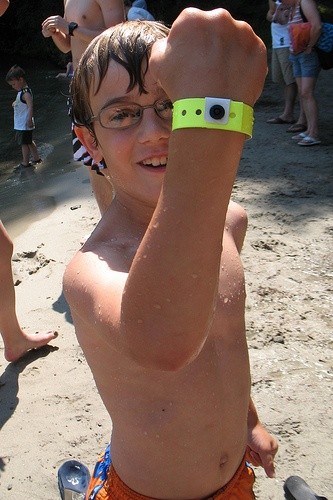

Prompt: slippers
290;132;308;139
283;475;329;500
286;122;307;132
57;460;91;500
297;136;322;146
267;117;295;124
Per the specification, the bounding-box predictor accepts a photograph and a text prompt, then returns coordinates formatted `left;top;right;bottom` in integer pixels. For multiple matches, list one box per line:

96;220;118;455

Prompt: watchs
68;22;78;36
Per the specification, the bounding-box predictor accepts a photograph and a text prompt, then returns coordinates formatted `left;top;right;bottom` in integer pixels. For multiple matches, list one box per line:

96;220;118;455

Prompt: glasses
88;97;175;130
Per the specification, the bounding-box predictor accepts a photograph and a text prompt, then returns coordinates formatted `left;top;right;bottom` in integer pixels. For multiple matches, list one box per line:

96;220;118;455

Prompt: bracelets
170;96;255;141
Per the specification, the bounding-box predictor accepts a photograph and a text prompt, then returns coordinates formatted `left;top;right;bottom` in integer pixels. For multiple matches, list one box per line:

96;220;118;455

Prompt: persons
6;67;42;170
56;54;73;79
0;219;58;362
283;0;322;145
63;8;279;500
42;0;127;215
266;0;307;133
125;0;155;21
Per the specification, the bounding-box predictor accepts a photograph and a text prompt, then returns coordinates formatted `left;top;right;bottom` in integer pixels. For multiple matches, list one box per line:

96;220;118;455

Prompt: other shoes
13;162;32;170
31;159;43;164
